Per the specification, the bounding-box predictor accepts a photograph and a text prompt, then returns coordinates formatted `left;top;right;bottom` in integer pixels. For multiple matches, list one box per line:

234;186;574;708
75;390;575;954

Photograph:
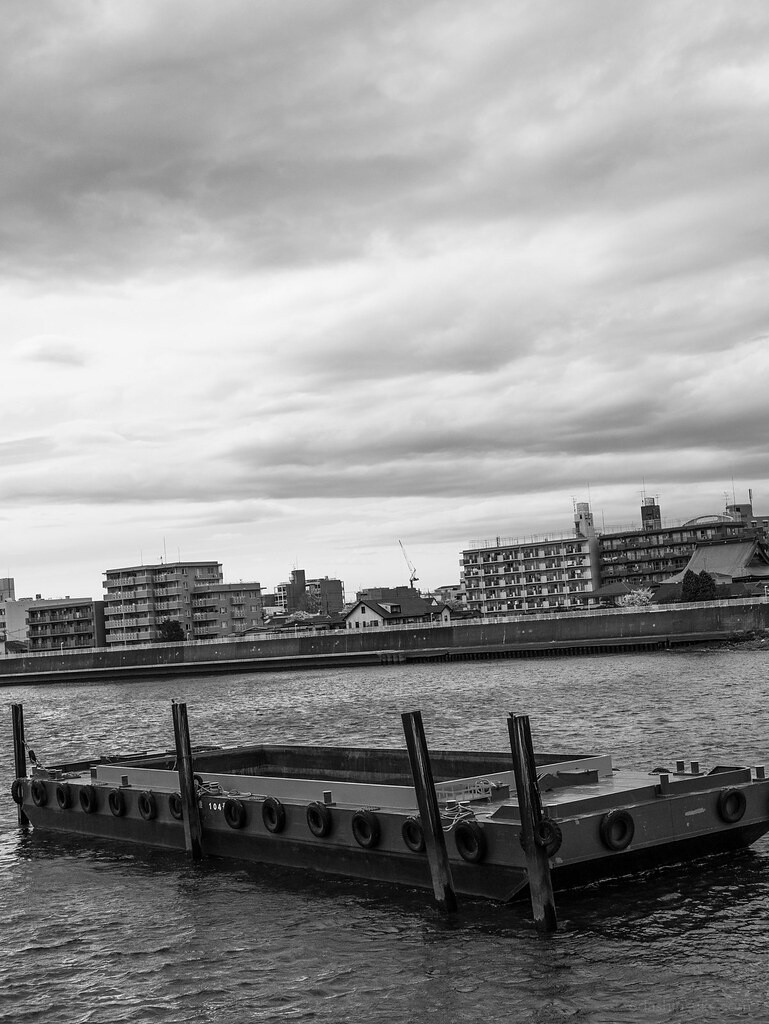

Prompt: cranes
399;540;419;588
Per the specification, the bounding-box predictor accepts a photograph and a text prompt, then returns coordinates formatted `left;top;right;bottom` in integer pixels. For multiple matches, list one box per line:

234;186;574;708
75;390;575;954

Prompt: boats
11;703;769;937
